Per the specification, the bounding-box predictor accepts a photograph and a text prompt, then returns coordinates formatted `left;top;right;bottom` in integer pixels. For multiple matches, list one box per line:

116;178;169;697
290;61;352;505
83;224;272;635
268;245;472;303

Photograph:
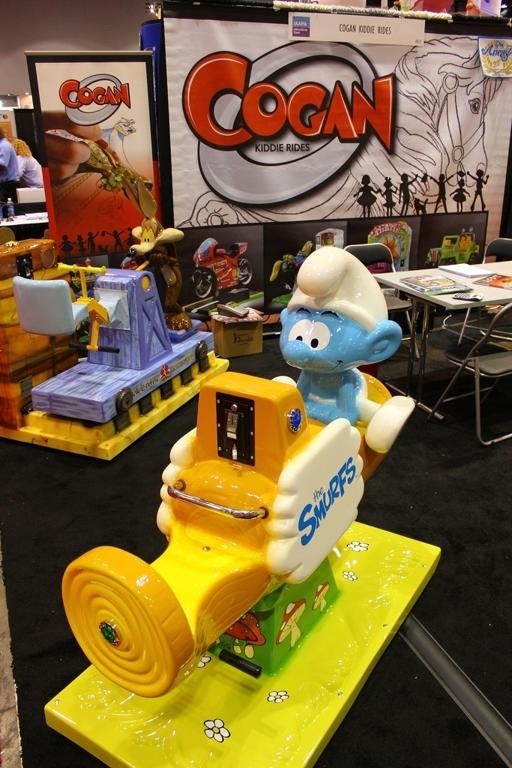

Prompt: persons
0;127;19;204
8;138;45;189
39;107;103;186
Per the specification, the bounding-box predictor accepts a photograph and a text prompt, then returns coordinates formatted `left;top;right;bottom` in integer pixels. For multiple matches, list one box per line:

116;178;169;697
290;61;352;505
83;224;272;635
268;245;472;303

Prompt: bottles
6;197;16;222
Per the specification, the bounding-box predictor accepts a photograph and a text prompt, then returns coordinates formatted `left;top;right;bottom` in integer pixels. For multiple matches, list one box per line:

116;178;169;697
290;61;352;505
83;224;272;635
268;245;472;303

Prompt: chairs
343;238;512;446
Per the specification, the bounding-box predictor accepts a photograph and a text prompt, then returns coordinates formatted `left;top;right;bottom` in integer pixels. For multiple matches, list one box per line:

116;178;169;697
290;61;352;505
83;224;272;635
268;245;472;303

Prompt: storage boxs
210;318;263;358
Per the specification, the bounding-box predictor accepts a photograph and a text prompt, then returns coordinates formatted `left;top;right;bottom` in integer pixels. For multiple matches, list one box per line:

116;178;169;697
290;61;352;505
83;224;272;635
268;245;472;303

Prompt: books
399;263;512;296
208;307;266;325
